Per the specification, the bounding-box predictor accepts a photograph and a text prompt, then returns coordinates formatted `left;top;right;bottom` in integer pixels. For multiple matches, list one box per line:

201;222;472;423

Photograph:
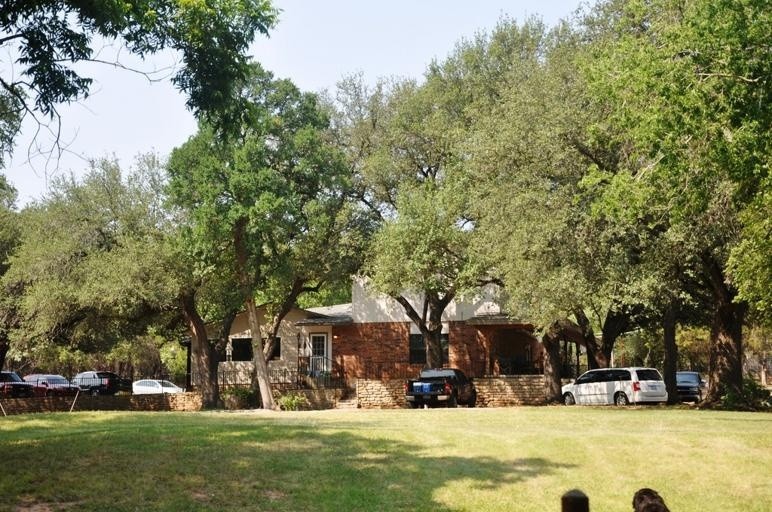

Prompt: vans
561;367;669;407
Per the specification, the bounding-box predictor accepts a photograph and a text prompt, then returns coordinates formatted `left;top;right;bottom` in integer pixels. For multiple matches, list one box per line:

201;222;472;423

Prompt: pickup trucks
404;367;477;409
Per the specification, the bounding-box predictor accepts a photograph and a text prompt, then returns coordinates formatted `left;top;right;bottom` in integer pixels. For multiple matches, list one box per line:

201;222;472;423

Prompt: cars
23;374;80;397
0;371;33;398
132;379;186;395
71;371;132;395
676;372;702;404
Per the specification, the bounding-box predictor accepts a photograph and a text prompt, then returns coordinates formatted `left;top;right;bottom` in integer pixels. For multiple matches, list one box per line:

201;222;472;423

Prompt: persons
560;488;590;512
632;487;670;512
